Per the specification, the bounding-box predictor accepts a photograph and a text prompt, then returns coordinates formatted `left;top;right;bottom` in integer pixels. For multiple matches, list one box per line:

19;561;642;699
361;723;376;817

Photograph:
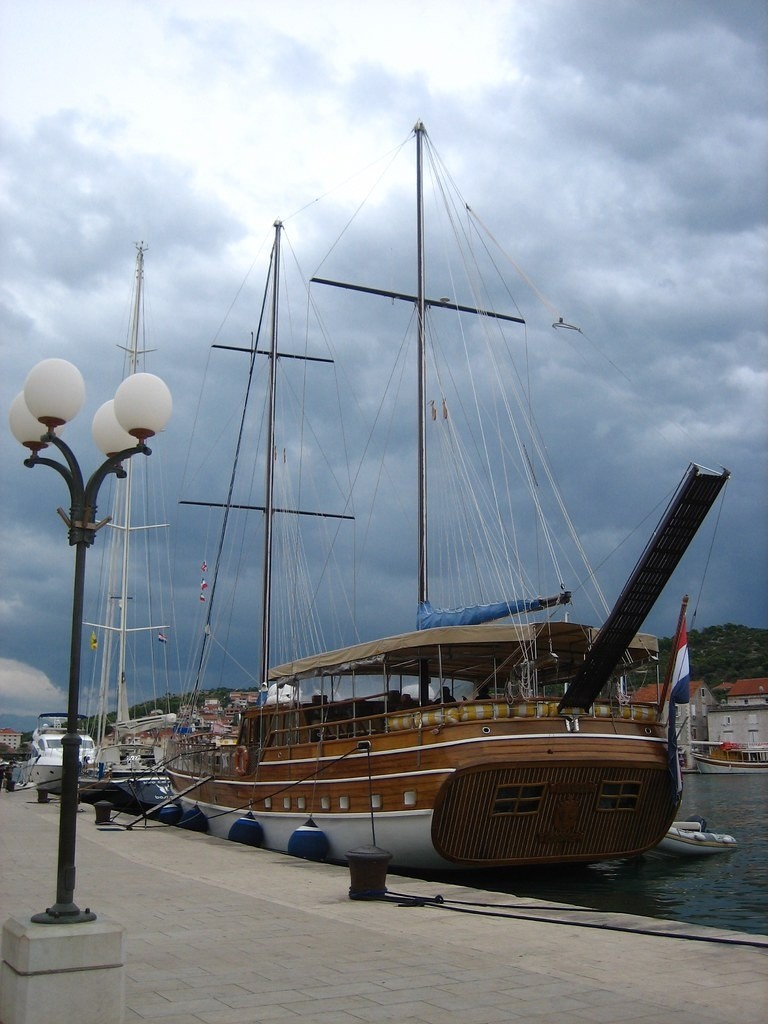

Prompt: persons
433;686;458;704
5;760;17;792
462;684;492;701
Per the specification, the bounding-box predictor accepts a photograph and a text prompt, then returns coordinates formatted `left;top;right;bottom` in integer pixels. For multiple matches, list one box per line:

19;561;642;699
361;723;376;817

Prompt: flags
158;631;167;645
201;561;209;573
200;576;207;590
200;593;206;604
91;630;97;650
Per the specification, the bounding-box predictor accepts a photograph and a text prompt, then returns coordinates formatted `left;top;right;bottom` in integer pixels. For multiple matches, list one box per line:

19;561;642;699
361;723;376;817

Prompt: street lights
11;356;175;930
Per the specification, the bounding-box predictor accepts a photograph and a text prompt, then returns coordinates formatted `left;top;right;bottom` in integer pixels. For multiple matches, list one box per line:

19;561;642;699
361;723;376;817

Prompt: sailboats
25;120;733;880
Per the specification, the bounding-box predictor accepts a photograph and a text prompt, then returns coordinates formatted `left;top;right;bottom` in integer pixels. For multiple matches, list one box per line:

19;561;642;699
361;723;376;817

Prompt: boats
652;813;739;858
692;738;767;776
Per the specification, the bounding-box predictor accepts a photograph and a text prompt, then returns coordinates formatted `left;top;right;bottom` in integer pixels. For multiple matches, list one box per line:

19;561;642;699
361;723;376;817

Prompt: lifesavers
235;746;249;776
